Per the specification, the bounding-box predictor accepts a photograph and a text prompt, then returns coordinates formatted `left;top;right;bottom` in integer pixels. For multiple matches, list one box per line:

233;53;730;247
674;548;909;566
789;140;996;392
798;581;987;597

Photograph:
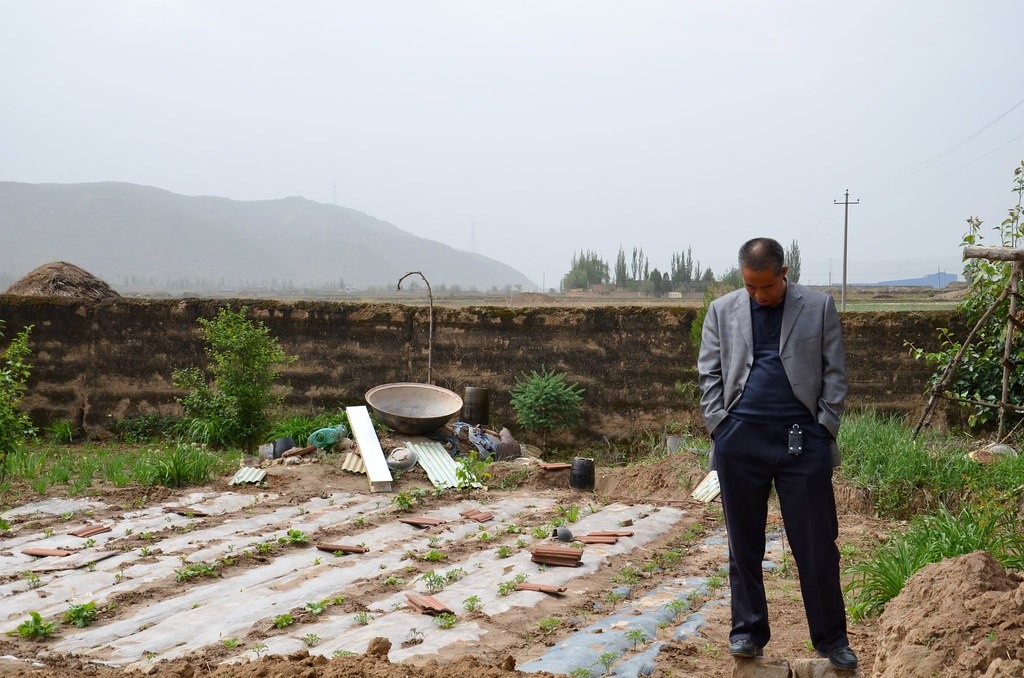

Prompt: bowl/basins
386;447;418;471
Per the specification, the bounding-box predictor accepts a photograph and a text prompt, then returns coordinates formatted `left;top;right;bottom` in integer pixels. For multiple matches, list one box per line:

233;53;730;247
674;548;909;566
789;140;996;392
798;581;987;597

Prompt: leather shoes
728;638;764;657
816;642;857;670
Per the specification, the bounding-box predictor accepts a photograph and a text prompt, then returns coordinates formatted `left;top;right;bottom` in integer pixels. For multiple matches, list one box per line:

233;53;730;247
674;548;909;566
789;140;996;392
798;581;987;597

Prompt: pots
364;382;463;434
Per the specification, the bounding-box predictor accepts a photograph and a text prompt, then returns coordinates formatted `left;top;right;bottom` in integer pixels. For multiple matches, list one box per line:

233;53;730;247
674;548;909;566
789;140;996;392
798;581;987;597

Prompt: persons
697;238;857;668
